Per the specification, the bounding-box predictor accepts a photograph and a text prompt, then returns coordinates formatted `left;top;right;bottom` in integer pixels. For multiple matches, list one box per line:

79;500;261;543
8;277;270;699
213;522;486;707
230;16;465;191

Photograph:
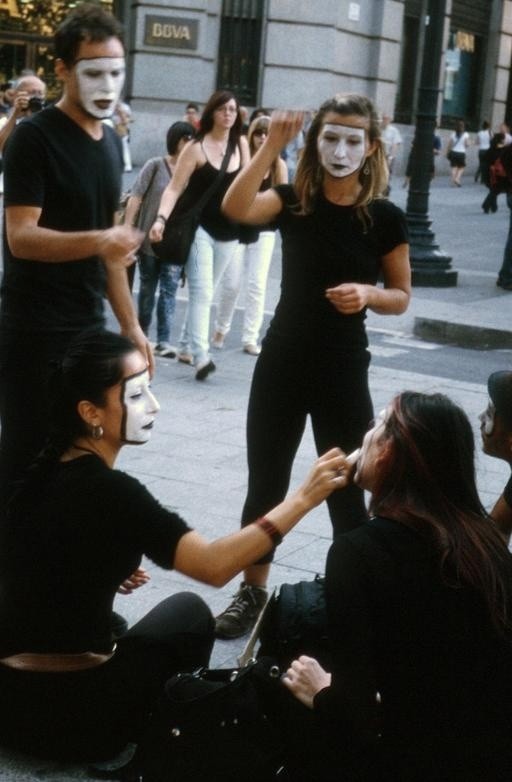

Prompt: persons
1;0;510;776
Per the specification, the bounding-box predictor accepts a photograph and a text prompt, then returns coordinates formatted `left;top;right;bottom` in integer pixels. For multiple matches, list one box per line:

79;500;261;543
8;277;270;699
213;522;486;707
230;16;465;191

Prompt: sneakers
155;339;261;381
214;582;269;640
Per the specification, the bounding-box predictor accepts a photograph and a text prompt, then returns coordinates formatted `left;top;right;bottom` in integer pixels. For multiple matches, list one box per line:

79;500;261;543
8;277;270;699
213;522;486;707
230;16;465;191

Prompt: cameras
26;96;47;114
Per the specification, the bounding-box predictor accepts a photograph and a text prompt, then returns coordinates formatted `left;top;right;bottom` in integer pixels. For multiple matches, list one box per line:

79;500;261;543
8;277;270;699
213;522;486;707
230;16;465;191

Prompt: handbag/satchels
136;663;289;782
489;159;506;190
149;213;200;262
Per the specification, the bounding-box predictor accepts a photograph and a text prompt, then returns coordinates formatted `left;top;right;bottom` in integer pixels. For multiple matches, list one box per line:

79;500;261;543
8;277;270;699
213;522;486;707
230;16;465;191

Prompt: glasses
253;129;268;137
